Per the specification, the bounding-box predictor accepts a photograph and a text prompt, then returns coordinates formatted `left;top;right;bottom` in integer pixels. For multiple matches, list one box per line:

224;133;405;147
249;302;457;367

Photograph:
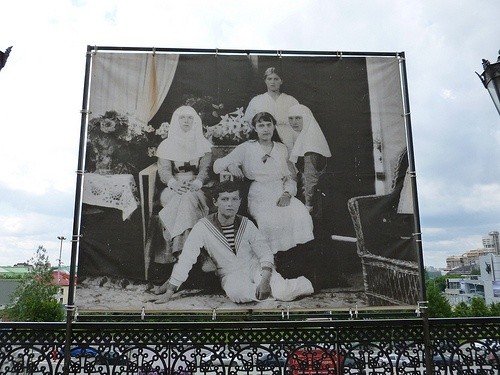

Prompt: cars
202;350;243;367
53;343;132;365
255;334;500;375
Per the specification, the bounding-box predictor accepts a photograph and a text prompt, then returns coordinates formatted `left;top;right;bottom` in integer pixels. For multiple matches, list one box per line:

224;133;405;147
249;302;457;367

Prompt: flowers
87;106;254;162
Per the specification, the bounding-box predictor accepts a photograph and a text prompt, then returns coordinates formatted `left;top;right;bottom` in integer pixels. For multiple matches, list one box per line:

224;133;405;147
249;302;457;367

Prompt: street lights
57;236;66;285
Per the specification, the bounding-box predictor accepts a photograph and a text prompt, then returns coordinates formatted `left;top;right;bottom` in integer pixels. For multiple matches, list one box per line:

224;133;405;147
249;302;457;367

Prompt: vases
95;142;113;174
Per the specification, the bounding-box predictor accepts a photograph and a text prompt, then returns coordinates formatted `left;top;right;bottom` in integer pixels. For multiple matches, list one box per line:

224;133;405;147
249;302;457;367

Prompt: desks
81;171;146;278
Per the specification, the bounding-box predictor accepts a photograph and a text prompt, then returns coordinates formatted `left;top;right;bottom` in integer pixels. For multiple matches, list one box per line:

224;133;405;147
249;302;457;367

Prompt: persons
287;104;330;268
244;66;299;151
212;112;315;279
148;181;349;304
155;105;216;273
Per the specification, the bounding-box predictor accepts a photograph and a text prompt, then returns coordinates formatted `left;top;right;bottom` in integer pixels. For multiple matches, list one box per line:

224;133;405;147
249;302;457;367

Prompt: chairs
138;146;239;280
348;146;422;307
